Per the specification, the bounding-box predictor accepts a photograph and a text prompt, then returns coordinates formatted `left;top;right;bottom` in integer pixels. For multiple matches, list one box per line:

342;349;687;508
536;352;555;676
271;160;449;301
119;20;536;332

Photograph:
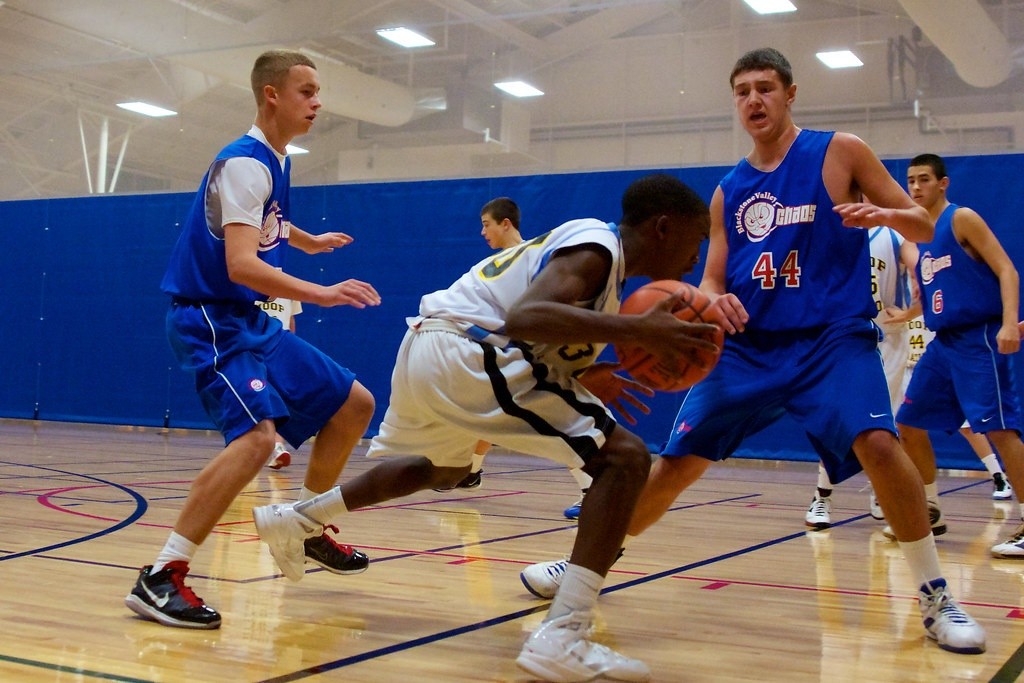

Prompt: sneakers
520;551;571;599
859;478;885;520
252;502;324;582
991;517;1024;559
804;491;834;530
432;469;484;493
992;471;1012;500
267;446;291;470
124;562;221;630
516;610;650;683
912;574;986;654
882;499;947;541
564;493;586;519
303;525;369;575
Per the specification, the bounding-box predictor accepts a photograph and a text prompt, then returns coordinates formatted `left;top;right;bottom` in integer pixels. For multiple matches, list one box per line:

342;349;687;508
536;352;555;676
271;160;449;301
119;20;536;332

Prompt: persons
125;50;375;630
804;153;1023;559
254;297;303;469
520;50;988;654
252;176;712;683
433;198;595;520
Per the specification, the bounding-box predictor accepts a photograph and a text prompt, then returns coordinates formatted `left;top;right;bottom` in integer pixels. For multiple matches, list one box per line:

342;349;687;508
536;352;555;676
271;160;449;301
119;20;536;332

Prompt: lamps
743;0;798;16
815;46;864;68
285;143;310;156
493;77;545;97
374;24;437;48
115;97;178;118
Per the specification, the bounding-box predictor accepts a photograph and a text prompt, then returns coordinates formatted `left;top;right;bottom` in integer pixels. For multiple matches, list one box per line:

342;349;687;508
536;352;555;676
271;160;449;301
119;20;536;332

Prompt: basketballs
613;281;725;392
